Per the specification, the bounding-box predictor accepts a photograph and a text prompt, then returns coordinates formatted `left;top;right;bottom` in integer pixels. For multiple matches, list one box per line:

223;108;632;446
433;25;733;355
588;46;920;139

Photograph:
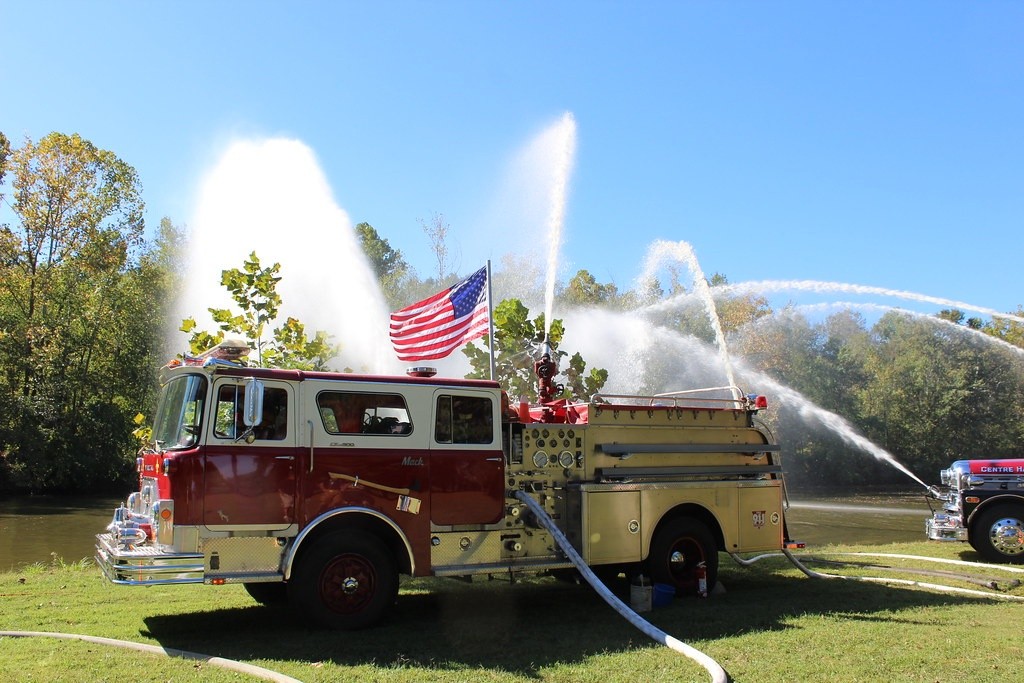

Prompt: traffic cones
520;395;530;423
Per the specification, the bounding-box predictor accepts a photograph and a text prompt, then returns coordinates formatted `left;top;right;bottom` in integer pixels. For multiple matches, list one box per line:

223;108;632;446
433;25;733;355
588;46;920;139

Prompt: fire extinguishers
695;560;707;598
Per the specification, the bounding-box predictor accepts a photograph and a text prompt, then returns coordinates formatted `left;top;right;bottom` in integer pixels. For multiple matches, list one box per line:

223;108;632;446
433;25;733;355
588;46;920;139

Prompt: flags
389;263;489;362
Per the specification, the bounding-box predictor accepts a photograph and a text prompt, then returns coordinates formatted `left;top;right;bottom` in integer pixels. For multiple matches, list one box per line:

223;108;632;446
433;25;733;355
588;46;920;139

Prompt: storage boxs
650;582;676;608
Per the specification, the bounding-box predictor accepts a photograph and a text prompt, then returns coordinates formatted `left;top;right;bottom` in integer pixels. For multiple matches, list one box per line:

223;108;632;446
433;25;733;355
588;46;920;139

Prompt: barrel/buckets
651;583;677;611
630;578;652;613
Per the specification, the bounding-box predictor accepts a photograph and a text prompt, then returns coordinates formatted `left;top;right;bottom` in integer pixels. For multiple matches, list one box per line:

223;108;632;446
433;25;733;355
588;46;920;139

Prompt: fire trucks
92;260;808;633
925;459;1024;564
